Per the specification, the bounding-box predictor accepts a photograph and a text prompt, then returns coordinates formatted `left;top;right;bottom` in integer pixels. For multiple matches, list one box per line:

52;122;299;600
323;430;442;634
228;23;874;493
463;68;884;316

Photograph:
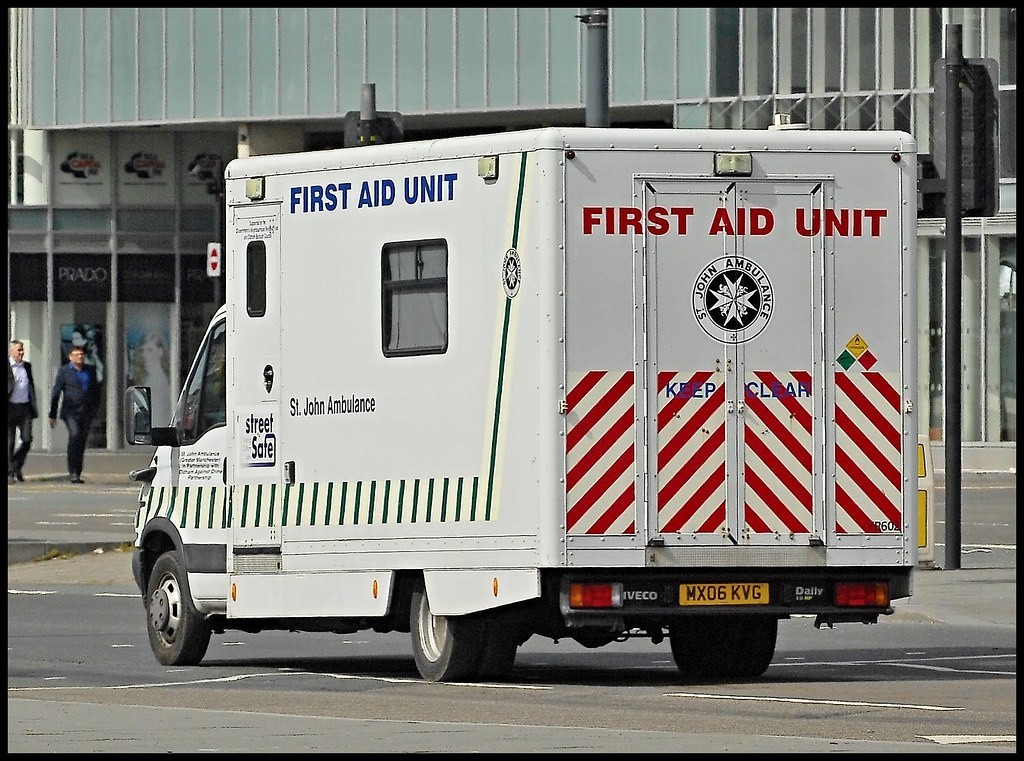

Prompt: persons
140;332;170;426
48;346;100;483
8;340;38;484
67;327;103;383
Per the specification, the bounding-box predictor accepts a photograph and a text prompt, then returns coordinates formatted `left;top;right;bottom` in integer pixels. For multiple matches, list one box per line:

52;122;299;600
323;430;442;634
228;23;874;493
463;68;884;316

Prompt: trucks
126;123;936;691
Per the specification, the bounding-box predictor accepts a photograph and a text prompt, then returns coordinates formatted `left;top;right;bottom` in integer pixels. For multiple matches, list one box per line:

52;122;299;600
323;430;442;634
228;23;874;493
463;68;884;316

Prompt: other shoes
15;470;24;482
70;473;85;483
8;475;14;485
